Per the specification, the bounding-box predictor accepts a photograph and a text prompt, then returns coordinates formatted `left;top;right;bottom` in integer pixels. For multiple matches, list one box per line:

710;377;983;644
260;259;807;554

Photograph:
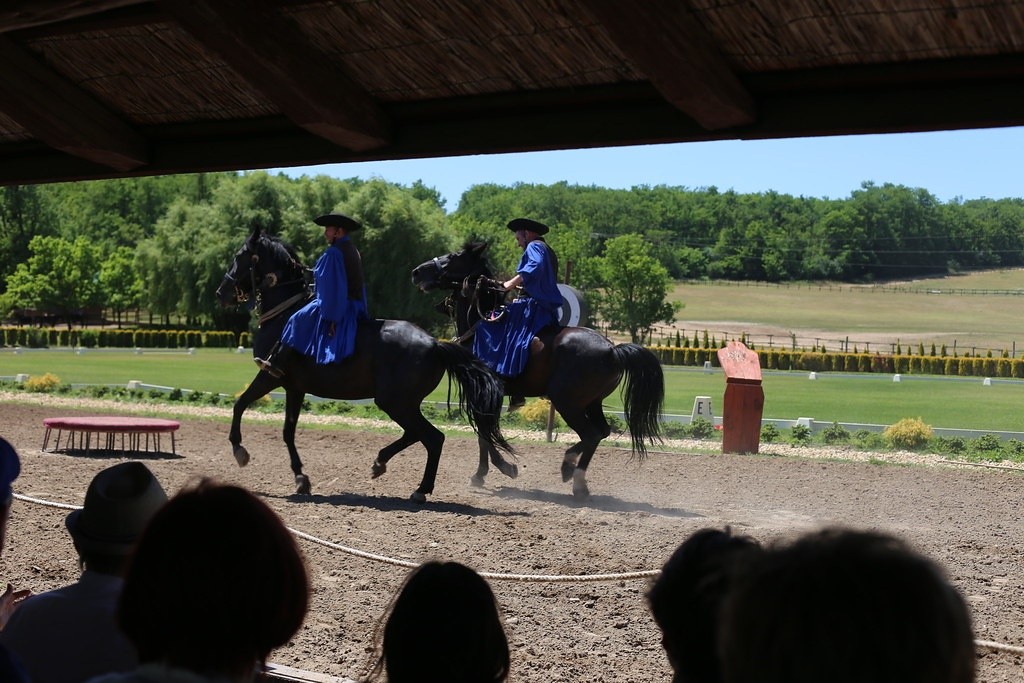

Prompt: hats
65;460;172;556
313;213;358;232
507;218;549;236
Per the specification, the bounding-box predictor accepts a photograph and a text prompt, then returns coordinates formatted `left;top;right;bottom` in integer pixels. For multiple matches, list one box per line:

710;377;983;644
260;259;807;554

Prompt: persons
0;438;168;683
646;530;763;683
472;218;563;412
86;485;307;683
384;562;510;683
253;213;369;381
719;531;976;683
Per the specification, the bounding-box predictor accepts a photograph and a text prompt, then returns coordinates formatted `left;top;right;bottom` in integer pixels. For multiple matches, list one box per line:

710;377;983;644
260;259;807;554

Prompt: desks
40;417;180;457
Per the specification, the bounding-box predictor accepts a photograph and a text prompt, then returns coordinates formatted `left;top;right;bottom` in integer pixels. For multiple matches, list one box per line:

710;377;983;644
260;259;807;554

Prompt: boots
253;343;293;379
506;372;526;412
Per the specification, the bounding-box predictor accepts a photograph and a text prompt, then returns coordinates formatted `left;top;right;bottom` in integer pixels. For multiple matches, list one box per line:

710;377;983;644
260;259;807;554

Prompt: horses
410;230;666;501
214;220;526;504
